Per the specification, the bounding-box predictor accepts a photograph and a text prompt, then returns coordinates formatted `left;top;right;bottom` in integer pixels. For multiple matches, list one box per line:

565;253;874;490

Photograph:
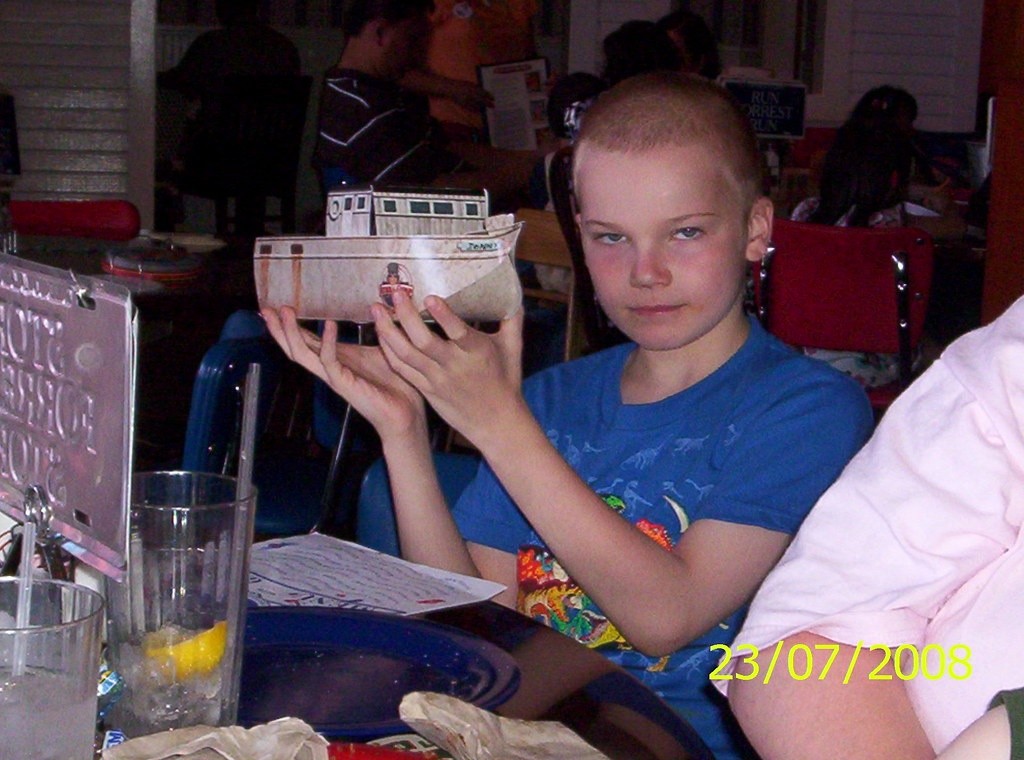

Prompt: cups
0;471;258;759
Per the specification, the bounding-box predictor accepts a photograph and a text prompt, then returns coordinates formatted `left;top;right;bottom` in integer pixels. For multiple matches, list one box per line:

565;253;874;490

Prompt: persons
311;2;520;198
158;0;303;234
534;73;604;289
791;86;969;394
662;10;724;76
708;297;1024;760
260;71;877;760
602;20;676;90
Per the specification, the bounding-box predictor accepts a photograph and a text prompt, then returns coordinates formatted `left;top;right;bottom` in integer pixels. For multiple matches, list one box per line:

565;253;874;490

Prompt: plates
237;606;521;742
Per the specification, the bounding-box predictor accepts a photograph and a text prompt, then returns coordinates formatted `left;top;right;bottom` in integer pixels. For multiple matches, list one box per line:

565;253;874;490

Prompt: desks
399;598;718;760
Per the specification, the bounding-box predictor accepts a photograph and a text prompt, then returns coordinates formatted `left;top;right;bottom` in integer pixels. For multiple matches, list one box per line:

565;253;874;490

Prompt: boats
252;186;525;328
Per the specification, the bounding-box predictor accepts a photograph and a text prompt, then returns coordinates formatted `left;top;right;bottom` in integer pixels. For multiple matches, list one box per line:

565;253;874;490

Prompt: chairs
182;311;365;540
470;206;582;365
750;216;935;405
